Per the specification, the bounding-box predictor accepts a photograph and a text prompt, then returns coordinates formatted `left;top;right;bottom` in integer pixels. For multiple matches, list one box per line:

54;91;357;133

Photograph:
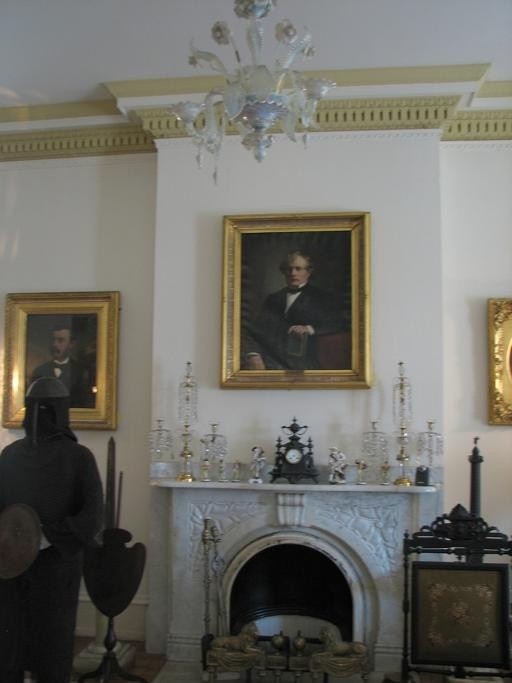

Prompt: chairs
383;504;512;683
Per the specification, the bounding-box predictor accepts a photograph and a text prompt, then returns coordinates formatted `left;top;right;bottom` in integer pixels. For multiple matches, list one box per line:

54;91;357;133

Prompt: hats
25;378;70;408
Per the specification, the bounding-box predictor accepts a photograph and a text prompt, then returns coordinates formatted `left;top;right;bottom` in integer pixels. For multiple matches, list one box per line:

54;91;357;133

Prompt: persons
0;378;104;683
241;250;348;371
28;325;94;408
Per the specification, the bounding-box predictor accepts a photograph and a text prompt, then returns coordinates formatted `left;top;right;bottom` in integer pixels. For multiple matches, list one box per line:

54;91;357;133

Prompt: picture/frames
1;289;120;430
487;299;512;426
219;211;370;389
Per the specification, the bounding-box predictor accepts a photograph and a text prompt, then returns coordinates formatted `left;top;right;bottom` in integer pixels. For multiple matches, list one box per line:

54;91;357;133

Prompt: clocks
268;417;321;483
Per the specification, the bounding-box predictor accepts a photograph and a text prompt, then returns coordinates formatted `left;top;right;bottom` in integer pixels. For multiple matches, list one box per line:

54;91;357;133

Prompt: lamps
167;0;338;185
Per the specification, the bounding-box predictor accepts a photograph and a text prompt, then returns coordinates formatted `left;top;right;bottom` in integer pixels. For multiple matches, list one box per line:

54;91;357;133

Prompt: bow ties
52;362;64;368
287;288;300;295
